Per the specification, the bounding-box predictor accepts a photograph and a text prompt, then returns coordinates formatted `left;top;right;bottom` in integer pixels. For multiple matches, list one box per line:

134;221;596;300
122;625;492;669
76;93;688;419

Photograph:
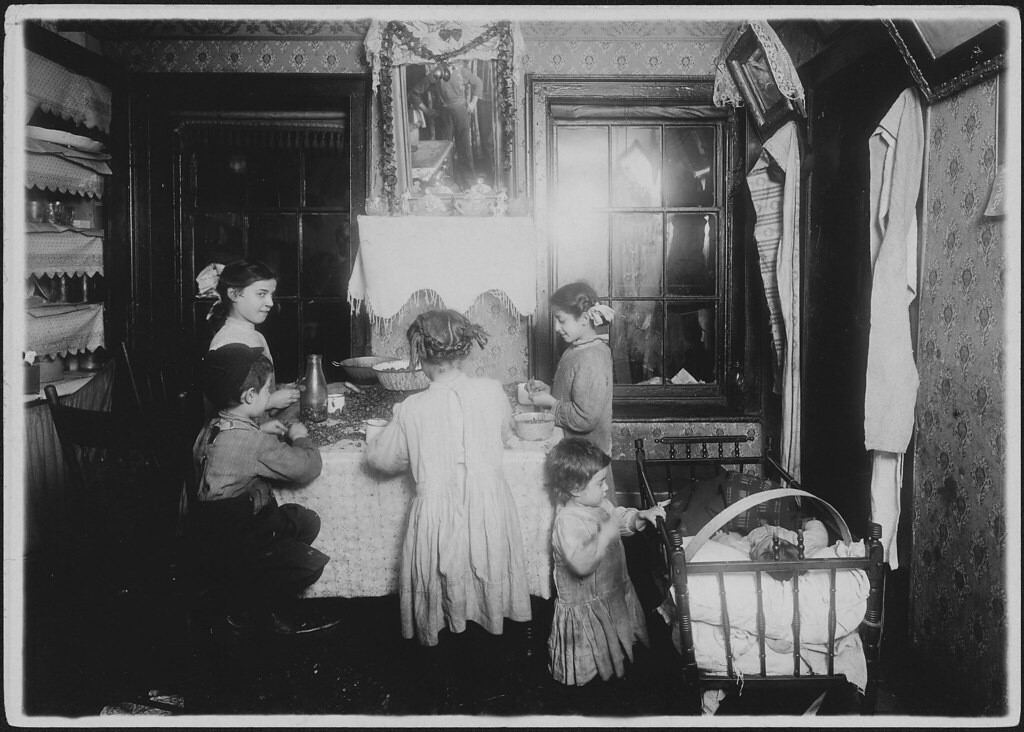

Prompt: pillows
668;533;870;645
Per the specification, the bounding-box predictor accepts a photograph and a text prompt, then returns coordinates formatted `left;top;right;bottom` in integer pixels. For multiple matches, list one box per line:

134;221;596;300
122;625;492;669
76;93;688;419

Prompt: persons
410;62;485;190
192;343;342;634
545;437;666;688
524;282;619;511
203;259;280;421
363;309;532;646
713;514;828;582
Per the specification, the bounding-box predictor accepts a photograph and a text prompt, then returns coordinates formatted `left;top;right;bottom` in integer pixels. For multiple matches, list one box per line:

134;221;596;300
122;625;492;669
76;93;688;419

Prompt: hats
202;342;265;408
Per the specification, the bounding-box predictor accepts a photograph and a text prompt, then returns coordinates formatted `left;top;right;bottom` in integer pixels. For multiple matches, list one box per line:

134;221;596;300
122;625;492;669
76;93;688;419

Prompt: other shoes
251;610;298;635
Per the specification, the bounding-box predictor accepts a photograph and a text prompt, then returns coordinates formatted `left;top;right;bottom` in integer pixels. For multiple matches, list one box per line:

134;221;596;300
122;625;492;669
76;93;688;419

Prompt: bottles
299;354;328;422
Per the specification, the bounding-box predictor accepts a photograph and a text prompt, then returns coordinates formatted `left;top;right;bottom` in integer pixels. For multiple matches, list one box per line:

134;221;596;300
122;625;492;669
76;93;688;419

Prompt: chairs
45;340;340;661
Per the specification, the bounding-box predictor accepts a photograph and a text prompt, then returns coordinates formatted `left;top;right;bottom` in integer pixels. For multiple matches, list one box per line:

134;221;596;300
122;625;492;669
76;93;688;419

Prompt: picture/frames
881;17;1008;103
714;19;810;144
372;17;517;216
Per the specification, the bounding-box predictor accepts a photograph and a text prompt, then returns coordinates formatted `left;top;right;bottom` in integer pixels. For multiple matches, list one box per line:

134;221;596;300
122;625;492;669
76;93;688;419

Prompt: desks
269;383;566;659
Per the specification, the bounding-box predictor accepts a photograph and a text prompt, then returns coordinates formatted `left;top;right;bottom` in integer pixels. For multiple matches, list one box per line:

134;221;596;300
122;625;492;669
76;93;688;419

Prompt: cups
59;205;74;225
45;202;61;224
365;418;389;444
328;394;345;414
29;201;44;222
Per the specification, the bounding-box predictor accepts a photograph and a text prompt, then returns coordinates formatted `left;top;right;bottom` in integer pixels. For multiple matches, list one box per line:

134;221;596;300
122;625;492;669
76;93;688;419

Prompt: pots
332;356;402;386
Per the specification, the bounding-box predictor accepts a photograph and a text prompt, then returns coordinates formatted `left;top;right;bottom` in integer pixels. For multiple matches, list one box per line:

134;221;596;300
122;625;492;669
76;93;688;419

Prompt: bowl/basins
514;413;555;440
372;359;429;391
517;383;550;406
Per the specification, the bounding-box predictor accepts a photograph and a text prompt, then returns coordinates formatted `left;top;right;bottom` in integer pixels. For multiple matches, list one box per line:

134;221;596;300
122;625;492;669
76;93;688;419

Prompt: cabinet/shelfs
25;25;119;496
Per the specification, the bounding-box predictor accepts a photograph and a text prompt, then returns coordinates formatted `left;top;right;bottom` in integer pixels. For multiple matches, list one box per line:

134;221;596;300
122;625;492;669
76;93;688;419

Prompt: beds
633;439;891;715
